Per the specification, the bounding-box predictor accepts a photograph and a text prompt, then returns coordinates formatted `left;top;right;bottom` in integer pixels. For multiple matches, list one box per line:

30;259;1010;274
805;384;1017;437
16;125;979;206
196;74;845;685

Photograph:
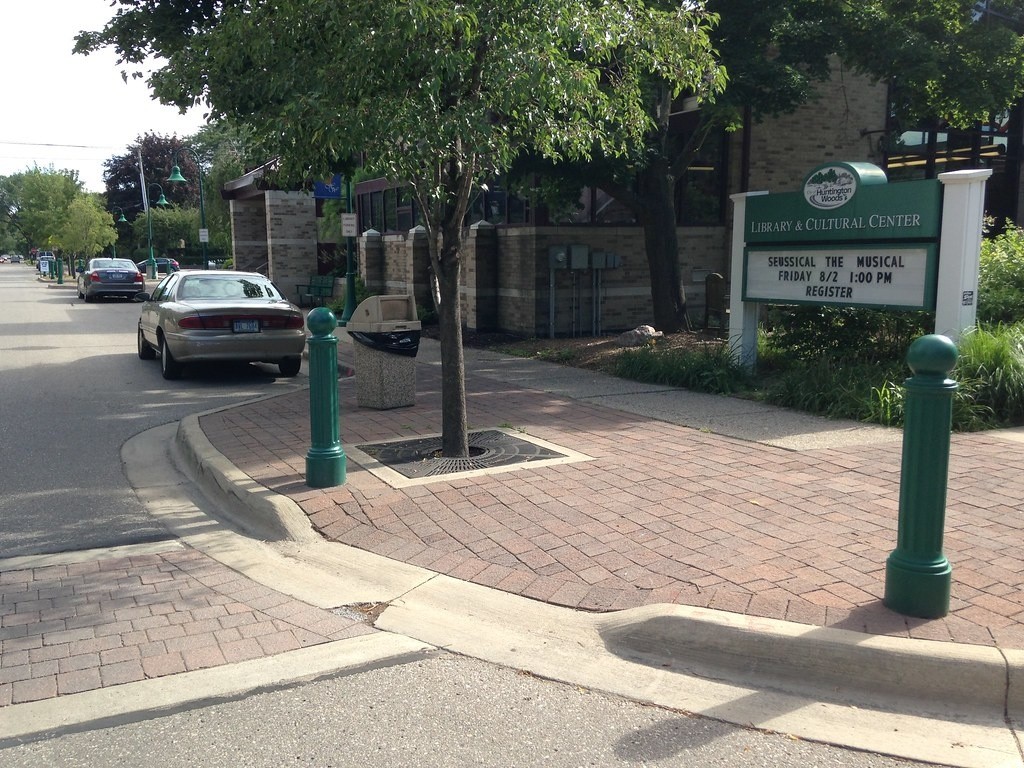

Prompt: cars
36;251;56;270
76;258;144;302
133;270;305;380
0;253;23;264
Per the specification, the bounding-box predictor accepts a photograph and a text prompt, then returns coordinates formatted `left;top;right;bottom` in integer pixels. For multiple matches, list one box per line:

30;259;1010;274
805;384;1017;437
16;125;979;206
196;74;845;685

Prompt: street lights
111;206;128;259
166;146;209;270
145;183;170;279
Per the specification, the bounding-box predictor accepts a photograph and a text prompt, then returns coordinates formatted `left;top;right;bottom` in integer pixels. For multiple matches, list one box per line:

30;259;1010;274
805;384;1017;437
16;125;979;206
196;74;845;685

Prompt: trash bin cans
344;294;421;409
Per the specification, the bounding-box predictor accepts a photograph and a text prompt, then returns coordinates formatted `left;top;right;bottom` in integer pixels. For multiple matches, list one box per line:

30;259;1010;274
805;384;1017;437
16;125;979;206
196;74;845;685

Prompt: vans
136;258;180;273
